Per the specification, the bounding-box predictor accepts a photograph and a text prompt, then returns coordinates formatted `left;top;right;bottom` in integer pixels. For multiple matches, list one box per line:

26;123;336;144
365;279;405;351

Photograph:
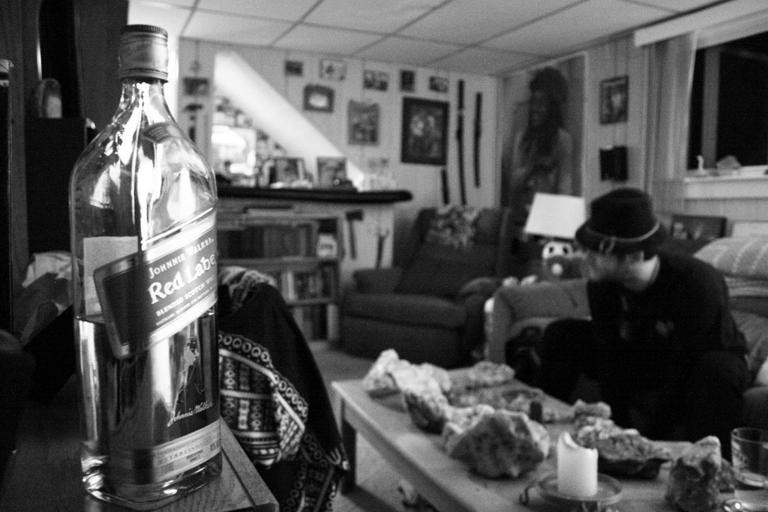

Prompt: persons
542;188;750;446
499;65;577;277
412;107;438;153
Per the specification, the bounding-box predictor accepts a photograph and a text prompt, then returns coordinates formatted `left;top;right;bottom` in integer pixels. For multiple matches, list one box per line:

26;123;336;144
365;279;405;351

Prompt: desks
210;186;416;347
2;366;278;511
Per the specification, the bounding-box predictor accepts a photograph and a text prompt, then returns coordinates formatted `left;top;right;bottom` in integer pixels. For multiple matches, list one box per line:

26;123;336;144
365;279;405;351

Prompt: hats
575;188;665;256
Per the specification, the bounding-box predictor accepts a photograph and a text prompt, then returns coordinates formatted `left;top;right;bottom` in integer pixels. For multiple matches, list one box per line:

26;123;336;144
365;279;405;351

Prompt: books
260;226;341;340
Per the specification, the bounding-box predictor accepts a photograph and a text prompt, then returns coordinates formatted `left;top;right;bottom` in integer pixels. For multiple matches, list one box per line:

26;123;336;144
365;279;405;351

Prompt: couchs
483;230;768;450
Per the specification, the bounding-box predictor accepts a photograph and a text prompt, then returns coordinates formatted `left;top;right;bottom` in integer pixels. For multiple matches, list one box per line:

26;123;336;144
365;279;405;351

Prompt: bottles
64;22;222;508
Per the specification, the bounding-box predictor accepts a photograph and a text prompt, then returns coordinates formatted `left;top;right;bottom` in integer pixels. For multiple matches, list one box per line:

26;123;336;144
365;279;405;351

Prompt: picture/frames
599;143;628;182
598;75;631;123
283;57;450;167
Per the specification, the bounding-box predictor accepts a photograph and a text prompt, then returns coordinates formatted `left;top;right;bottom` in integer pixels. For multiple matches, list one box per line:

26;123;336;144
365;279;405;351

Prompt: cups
730;428;768;511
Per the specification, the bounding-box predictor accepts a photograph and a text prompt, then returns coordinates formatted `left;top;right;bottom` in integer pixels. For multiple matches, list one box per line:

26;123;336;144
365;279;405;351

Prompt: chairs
336;205;522;352
